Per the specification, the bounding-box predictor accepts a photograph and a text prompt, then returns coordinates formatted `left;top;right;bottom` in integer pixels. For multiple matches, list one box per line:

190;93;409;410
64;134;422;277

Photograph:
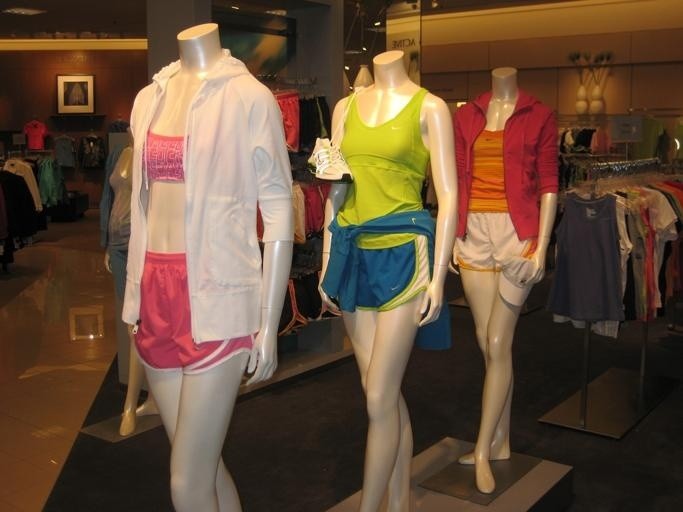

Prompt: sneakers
308;138;355;184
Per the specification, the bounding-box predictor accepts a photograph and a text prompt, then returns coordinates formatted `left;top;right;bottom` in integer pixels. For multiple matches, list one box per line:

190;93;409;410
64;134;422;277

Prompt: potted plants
566;48;614;113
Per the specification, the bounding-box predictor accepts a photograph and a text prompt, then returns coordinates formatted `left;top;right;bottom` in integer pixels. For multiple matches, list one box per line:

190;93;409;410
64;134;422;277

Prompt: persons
120;20;295;512
311;48;460;511
94;124;160;438
449;65;561;497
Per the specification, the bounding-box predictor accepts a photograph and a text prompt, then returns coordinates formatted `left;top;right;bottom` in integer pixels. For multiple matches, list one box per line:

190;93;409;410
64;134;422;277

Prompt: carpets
41;274;683;509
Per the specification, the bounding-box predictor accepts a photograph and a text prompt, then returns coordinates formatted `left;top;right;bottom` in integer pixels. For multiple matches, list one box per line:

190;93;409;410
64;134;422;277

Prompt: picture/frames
55;73;95;115
